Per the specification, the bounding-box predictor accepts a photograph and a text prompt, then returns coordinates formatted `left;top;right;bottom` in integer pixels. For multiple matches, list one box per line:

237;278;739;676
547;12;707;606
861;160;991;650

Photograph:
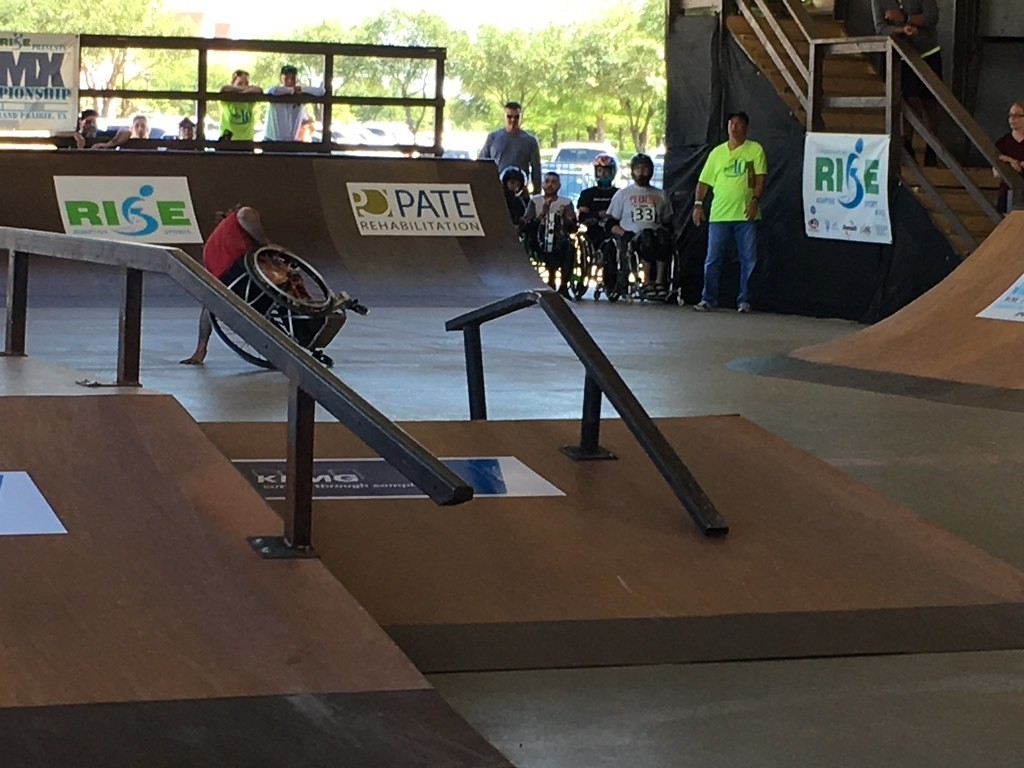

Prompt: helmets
593;153;617;184
630;153;654;182
499;165;528;197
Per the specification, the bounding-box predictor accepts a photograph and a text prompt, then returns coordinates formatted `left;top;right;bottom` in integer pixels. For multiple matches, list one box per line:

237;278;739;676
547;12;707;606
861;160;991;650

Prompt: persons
992;100;1024;217
179;203;286;365
832;0;942;166
52;63;326;150
691;111;768;312
477;101;674;295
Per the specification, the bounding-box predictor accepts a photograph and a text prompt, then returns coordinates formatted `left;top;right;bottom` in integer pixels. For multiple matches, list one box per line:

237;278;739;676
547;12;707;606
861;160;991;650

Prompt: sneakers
693;300;713;312
737;303;751;313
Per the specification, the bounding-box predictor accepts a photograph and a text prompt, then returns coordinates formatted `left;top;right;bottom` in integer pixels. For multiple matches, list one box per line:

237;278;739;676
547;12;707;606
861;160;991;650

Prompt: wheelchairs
520;230;685;307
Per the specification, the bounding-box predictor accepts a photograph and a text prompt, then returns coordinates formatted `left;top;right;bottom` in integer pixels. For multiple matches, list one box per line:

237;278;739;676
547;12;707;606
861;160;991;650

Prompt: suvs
551;143;622;187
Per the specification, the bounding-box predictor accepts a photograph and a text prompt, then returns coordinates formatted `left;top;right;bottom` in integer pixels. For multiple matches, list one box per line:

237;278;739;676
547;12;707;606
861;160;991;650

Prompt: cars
90;115;488;159
645;149;665;189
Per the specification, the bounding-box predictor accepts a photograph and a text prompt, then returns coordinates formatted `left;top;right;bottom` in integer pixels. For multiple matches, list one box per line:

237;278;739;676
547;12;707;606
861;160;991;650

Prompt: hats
80;109;98;120
179;117;196;127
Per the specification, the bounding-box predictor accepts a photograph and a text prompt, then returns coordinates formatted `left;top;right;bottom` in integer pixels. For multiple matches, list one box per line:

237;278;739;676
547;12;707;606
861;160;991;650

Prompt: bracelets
752;195;760;203
903;13;908;23
694;200;703;205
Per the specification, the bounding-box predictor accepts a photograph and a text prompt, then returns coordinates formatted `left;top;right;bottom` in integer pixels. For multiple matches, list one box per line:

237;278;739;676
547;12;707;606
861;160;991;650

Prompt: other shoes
604;287;622;302
642;283;656;295
546;282;557;290
654;282;668;295
903;138;920;165
558;286;573;301
923;143;938;167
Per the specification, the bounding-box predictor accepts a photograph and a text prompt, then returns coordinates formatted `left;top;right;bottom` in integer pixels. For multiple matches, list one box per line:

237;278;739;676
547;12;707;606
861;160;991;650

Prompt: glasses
231;70;249;84
1008;112;1024;118
507;114;520;119
82;120;97;124
